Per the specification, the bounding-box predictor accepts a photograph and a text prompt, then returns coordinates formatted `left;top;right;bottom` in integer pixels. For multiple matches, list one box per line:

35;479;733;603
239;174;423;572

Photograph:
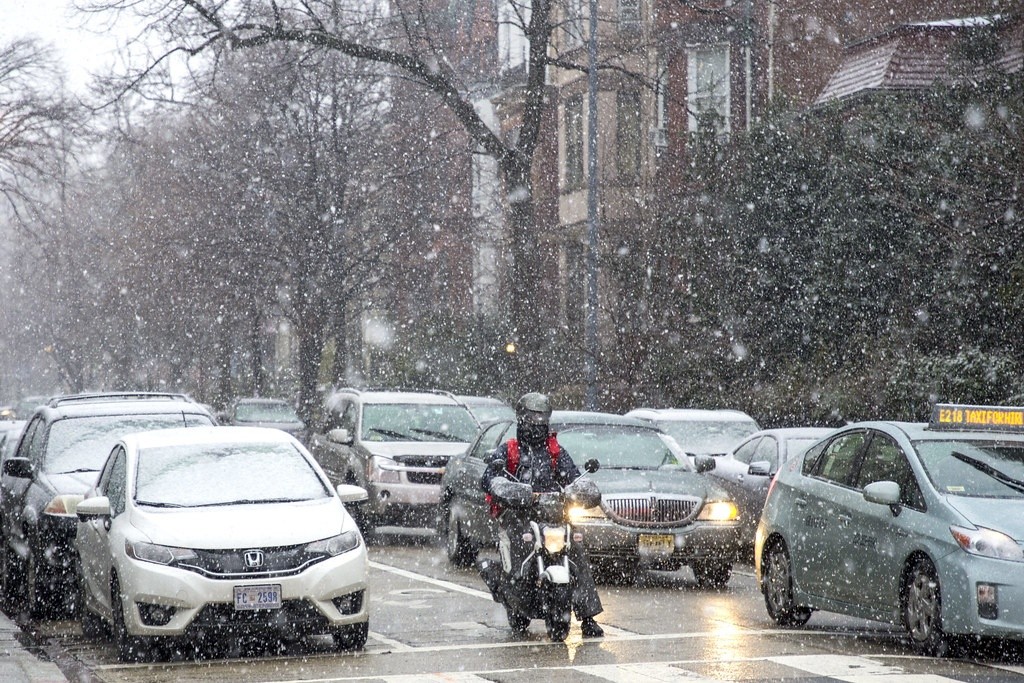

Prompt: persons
481;392;605;636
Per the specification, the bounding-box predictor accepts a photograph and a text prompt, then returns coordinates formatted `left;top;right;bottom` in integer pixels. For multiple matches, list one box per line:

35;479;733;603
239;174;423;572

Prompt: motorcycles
475;450;603;641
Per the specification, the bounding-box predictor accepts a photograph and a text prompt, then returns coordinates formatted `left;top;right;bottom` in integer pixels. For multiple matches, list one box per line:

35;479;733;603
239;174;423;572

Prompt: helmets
515;392;553;442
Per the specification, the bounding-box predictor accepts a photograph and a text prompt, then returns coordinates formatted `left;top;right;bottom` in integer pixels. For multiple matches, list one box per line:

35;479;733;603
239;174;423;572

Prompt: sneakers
581;619;604;638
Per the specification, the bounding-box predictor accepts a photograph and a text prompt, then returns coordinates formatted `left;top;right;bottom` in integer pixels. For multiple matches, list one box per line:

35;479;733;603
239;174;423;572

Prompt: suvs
0;391;219;621
308;388;487;544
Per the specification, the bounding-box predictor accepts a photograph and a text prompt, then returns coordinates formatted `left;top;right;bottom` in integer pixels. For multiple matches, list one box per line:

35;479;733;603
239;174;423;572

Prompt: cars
71;425;371;656
753;401;1024;654
710;426;839;547
623;409;762;468
218;397;309;444
1;420;28;476
457;395;516;426
439;411;742;588
